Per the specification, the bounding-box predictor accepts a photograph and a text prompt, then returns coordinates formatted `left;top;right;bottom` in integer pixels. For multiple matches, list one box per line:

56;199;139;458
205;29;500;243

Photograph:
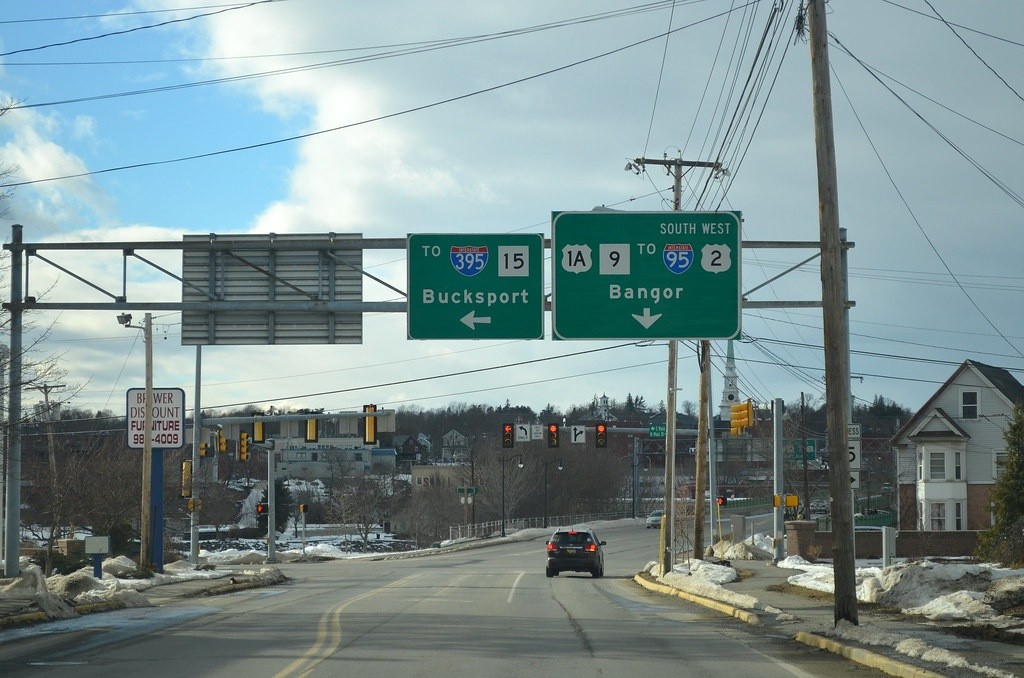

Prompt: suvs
545;527;607;578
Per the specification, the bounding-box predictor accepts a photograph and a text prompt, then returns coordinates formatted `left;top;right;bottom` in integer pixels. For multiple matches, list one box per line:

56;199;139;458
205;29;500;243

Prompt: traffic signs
516;425;531;441
552;209;743;340
407;233;543;341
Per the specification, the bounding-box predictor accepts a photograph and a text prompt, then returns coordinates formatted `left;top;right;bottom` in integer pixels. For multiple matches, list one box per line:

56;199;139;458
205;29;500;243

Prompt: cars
809;499;831;514
646;510;663;529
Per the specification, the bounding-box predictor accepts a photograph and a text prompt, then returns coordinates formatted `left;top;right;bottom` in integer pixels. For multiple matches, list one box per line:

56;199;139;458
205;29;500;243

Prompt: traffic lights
730;401;752;435
257;505;265;512
717;496;727;506
300;505;308;513
596;424;607;448
200;442;208;456
220;437;228;451
240;433;249;461
502;423;515;448
548;423;559;447
773;495;780;508
